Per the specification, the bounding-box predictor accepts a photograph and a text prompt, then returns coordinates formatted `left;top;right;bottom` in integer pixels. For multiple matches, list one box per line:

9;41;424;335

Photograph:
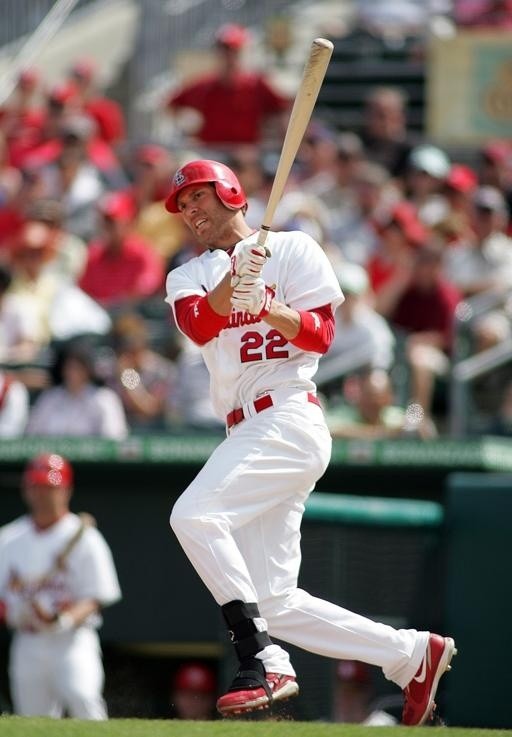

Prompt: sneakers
402;632;458;723
217;670;301;717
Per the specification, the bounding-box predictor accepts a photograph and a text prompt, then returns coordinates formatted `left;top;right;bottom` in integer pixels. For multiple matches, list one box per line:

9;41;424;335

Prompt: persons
175;662;214;720
0;453;124;721
165;160;459;727
333;658;399;728
0;24;510;440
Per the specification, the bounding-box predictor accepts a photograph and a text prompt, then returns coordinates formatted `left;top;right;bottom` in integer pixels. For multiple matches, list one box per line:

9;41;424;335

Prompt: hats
175;667;214;694
334;660;367;681
209;24;245;52
334;263;369;295
305;91;508;247
7;63;163;255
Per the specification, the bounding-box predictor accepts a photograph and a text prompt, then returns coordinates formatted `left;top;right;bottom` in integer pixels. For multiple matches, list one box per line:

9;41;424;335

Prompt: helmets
163;159;248;216
19;450;74;489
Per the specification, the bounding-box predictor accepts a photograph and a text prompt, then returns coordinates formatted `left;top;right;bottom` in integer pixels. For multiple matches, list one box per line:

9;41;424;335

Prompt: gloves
230;275;275;322
227;244;269;278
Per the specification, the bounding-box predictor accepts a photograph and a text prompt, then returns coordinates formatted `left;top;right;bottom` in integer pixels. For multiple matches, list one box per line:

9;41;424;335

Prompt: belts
225;392;324;427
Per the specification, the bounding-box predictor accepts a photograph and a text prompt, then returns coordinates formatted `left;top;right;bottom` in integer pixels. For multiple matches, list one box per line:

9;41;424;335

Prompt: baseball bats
33;512;97;612
260;36;334;243
